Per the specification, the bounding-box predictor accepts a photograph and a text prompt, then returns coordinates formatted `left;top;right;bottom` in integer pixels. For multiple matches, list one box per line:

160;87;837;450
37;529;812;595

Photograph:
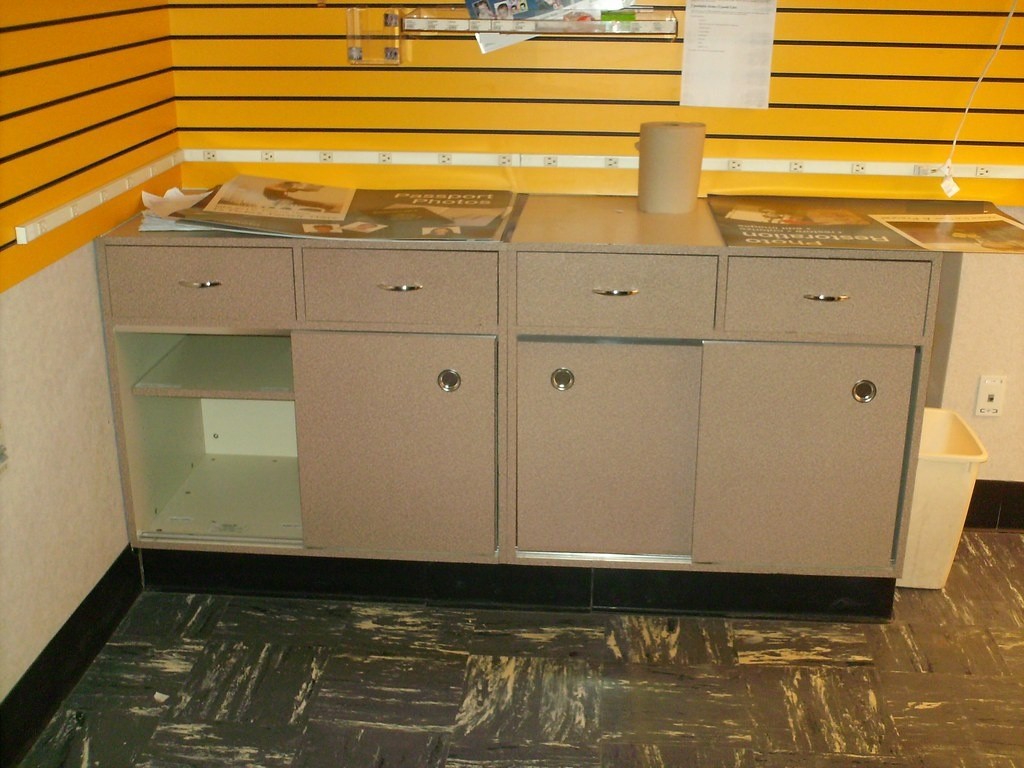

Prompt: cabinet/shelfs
91;187;524;624
507;193;946;625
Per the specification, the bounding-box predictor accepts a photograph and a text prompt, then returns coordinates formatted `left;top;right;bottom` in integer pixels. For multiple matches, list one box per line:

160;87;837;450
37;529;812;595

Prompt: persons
312;219;377;237
425;227;453;236
474;1;526;19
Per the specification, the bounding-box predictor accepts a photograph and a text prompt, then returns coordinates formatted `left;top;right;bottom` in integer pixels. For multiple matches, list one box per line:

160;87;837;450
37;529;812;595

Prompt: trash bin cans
895;406;990;592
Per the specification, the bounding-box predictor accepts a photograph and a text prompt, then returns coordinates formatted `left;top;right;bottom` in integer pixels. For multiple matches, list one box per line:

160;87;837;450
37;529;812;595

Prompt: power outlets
851;162;868;175
789;161;806;173
544;156;559;167
261;151;275;162
975;164;991;178
974;374;1007;416
438;152;452;165
605;157;620;168
203;151;217;161
319;151;334;163
727;159;744;171
378;153;392;164
498;155;512;166
